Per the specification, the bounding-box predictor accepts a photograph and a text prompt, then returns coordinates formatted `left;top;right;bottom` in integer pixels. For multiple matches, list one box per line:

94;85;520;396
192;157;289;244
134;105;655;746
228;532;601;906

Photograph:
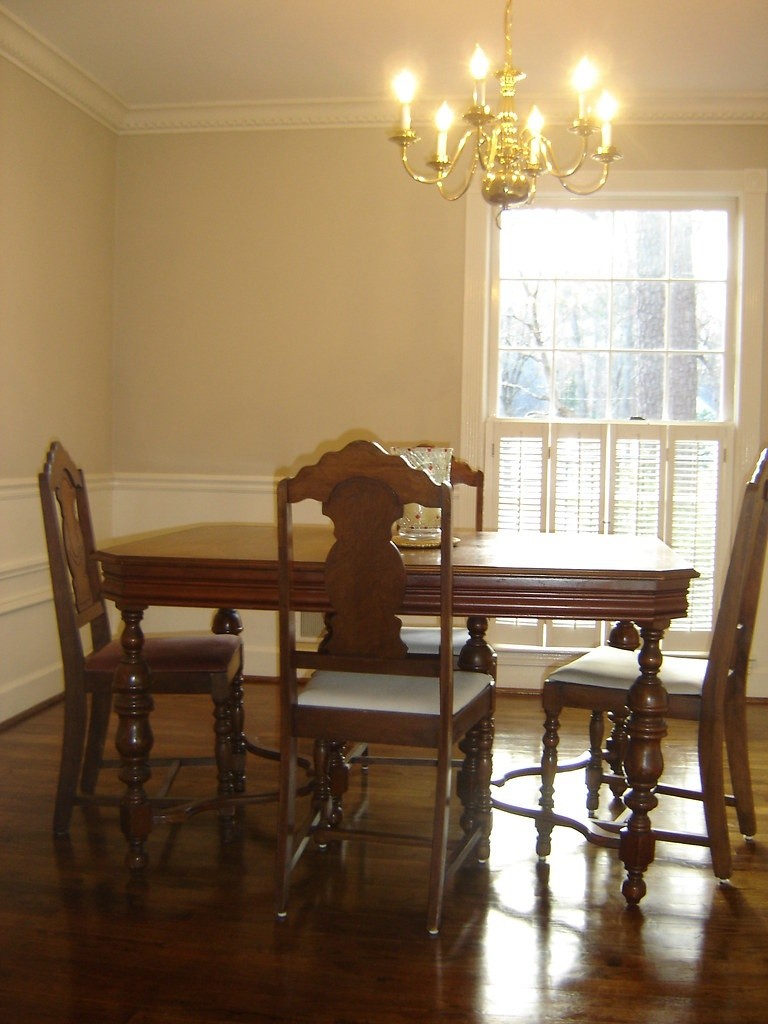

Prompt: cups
391;446;454;540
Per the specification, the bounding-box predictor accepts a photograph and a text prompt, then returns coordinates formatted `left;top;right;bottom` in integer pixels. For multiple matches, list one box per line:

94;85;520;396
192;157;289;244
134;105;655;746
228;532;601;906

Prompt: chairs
537;447;767;879
276;439;499;934
39;441;246;848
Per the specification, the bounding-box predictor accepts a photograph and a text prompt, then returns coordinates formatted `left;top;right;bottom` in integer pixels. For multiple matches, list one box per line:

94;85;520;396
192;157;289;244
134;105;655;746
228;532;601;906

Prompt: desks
93;521;700;907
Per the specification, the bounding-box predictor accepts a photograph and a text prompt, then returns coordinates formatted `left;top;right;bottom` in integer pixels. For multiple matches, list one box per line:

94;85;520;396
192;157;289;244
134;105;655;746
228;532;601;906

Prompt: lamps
389;0;623;231
